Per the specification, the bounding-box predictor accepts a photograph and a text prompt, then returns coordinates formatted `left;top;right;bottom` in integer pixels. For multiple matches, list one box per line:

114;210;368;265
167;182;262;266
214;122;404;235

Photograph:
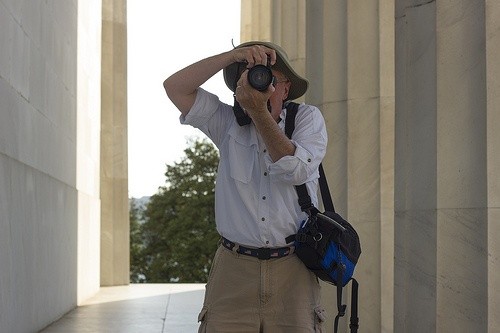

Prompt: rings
236;85;242;89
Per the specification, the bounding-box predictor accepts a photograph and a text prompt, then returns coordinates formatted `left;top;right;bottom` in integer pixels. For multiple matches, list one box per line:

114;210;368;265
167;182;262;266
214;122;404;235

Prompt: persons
163;41;328;333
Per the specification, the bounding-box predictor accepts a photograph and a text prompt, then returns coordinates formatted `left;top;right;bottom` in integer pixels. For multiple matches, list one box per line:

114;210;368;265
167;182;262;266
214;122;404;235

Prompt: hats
223;41;309;99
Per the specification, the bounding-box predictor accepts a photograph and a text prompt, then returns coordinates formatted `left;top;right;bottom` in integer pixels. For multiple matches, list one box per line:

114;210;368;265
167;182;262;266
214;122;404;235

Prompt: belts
220;236;296;259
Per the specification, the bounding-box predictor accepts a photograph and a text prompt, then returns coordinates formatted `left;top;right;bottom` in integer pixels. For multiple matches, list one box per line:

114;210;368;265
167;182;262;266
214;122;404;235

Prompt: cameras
243;56;274;91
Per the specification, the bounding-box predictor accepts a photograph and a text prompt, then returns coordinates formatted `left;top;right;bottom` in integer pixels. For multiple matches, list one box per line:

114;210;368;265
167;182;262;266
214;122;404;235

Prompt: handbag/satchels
295;211;362;285
